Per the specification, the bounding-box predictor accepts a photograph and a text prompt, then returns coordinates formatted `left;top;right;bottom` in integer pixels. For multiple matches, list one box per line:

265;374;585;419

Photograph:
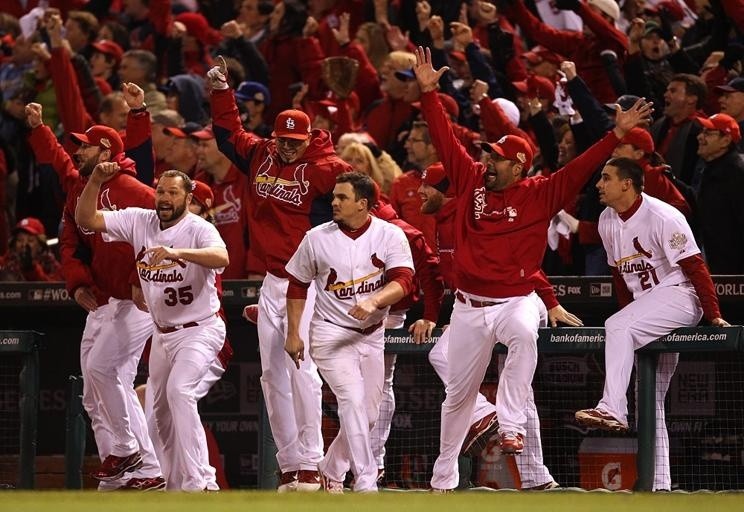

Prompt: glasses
405;138;424;143
698;128;717;138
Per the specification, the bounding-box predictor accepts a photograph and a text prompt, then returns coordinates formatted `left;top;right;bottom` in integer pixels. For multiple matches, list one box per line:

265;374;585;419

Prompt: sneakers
117;476;166;491
297;470;322;491
460;411;499;458
575;408;629;434
323;474;344;495
501;432;524;456
92;452;144;483
276;471;298;493
350;470;385;491
523;481;560;491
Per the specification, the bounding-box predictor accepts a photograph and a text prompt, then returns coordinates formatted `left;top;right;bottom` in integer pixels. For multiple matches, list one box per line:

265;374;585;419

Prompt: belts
323;318;383;336
455;291;508;308
153;321;199;333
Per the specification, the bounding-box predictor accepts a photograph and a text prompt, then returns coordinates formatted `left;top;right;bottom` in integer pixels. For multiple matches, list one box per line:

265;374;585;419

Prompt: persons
58;124;167;492
1;1;744;283
283;170;416;493
135;180;235;490
575;156;730;492
74;162;230;492
408;44;655;494
207;53;355;492
243;199;446;492
418;162;585;492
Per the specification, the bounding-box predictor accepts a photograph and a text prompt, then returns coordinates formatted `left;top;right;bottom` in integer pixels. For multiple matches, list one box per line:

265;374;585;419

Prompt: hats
233;80;270;106
271;109;312;140
394;44;571;195
92;40;124;61
163;121;203;141
603;22;744;155
191;124;215;141
157;80;177;93
14;217;45;238
191;179;215;212
70;124;123;157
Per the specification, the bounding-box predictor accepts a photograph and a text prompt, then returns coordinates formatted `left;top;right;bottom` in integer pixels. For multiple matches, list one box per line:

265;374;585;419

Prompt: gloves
207;55;230;90
